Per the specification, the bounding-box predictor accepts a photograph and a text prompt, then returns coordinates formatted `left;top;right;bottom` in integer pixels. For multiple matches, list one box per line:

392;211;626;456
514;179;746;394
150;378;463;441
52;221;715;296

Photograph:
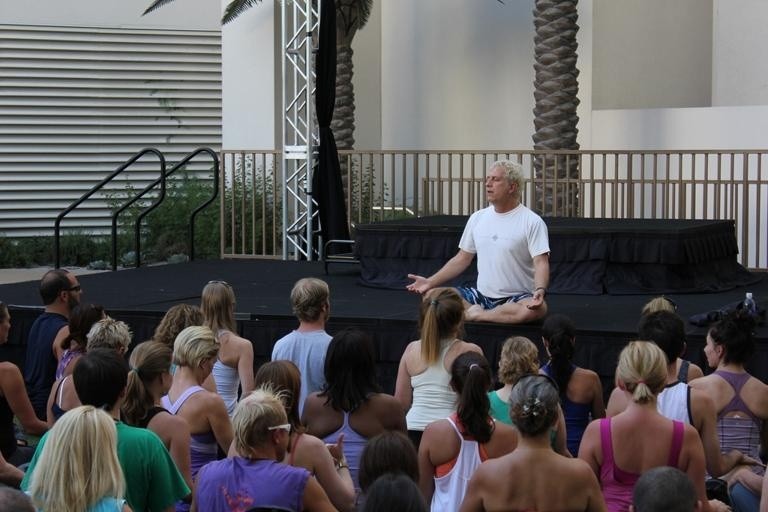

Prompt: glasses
71;285;81;292
268;424;291;434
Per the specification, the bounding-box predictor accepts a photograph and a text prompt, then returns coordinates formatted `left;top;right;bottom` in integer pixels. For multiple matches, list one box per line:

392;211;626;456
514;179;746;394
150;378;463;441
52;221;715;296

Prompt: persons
404;159;552;325
1;269;768;511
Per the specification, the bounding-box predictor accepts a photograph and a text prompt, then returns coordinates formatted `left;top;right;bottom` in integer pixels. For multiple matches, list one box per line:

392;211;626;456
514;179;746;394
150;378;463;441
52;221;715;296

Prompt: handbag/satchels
689;300;743;327
